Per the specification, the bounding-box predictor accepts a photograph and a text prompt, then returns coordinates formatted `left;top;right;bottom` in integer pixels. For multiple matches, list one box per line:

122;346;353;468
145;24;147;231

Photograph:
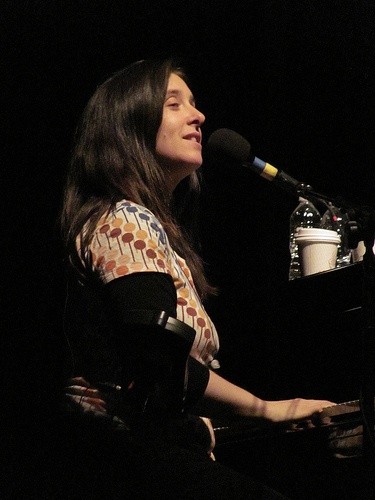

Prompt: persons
58;60;340;500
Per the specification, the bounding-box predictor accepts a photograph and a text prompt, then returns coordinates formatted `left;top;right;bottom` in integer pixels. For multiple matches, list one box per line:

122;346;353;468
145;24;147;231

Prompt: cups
295;228;340;277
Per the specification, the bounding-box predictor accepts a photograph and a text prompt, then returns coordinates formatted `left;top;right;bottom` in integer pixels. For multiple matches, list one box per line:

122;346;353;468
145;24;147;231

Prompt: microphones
206;128;306;196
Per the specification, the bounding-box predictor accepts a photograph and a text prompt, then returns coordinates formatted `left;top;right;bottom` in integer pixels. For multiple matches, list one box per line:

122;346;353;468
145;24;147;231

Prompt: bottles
322;201;342;268
289;183;321;280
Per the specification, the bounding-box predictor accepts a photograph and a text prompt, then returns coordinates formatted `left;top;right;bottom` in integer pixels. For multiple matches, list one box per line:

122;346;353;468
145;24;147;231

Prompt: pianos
203;258;375;500
